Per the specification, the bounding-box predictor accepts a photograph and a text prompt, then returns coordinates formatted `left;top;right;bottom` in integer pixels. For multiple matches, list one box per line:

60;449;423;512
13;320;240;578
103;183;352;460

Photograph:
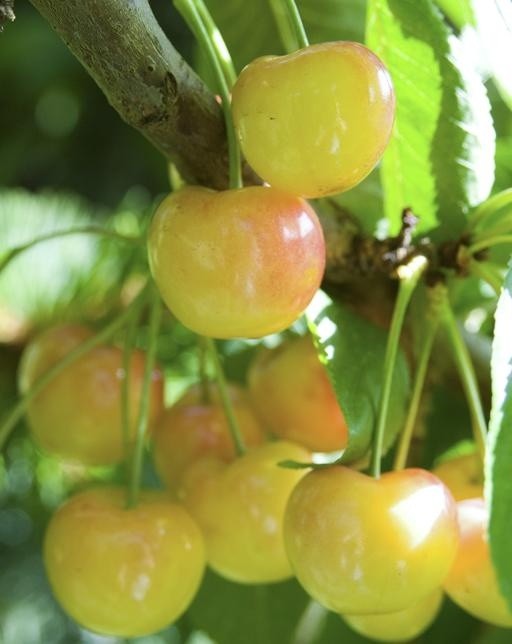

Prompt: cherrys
18;289;511;642
147;0;327;339
232;0;396;201
